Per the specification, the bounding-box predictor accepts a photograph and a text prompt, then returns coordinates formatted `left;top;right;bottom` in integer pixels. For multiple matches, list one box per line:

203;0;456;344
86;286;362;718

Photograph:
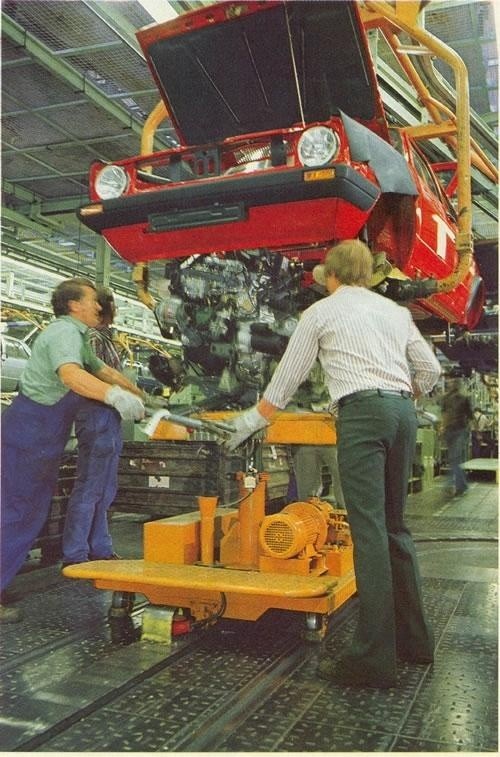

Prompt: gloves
104;384;145;422
215;408;270;451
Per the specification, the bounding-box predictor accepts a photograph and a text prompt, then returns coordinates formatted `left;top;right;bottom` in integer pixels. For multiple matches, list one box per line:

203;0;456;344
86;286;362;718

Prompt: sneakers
0;604;23;624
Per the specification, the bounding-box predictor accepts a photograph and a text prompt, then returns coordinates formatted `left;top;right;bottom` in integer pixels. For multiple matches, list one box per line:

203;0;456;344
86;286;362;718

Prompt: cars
79;0;488;339
1;334;33;394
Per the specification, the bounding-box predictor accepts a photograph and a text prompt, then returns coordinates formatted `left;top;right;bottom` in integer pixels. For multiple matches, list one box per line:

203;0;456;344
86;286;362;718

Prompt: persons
0;278;168;625
434;374;484;499
270;307;348;512
212;236;443;688
60;285;126;567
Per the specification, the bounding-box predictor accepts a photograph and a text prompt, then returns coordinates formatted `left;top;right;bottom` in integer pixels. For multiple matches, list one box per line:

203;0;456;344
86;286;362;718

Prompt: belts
337;388;415;406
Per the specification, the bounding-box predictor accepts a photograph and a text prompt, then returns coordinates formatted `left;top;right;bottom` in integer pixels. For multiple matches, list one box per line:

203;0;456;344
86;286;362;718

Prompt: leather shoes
396;644;434;664
319;658;396;691
